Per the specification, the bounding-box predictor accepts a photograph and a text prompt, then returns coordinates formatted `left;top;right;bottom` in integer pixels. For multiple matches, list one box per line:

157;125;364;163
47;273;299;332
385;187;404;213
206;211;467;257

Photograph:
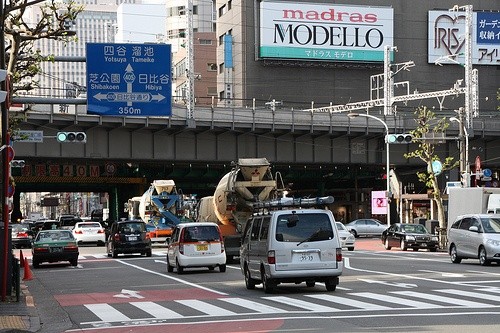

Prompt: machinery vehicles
192;158;291;265
123;180;198;243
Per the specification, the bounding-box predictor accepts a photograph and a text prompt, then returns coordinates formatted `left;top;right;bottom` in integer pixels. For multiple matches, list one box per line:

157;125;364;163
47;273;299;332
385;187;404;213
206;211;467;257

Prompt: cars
381;223;439;252
31;229;80;267
335;222;356;251
71;221;106;247
344;218;391;239
446;214;500;266
103;220;152;259
9;213;84;250
166;222;227;274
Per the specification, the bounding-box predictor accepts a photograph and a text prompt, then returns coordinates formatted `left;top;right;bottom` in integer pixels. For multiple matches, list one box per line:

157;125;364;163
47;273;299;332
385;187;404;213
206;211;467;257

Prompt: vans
235;208;344;295
91;208;109;227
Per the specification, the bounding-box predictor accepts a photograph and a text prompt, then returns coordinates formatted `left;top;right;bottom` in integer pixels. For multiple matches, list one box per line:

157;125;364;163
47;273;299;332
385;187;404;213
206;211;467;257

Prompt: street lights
347;113;391;228
448;117;468;173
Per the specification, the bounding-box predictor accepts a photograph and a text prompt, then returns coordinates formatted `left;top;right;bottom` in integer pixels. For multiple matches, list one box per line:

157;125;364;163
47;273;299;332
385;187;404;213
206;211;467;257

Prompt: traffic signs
85;41;172;117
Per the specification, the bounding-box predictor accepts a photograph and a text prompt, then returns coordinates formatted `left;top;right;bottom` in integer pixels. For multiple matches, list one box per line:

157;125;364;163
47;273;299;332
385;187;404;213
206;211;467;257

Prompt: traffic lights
56;132;88;143
10;160;26;168
0;69;8;102
387;133;413;144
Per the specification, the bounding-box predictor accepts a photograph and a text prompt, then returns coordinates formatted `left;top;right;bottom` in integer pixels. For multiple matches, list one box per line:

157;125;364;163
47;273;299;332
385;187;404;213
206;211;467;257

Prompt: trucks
447;186;500;240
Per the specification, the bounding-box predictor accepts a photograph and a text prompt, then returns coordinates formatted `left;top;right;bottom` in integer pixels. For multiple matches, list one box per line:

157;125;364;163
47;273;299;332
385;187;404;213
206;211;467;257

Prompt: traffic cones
19;249;25;267
23;257;33;281
